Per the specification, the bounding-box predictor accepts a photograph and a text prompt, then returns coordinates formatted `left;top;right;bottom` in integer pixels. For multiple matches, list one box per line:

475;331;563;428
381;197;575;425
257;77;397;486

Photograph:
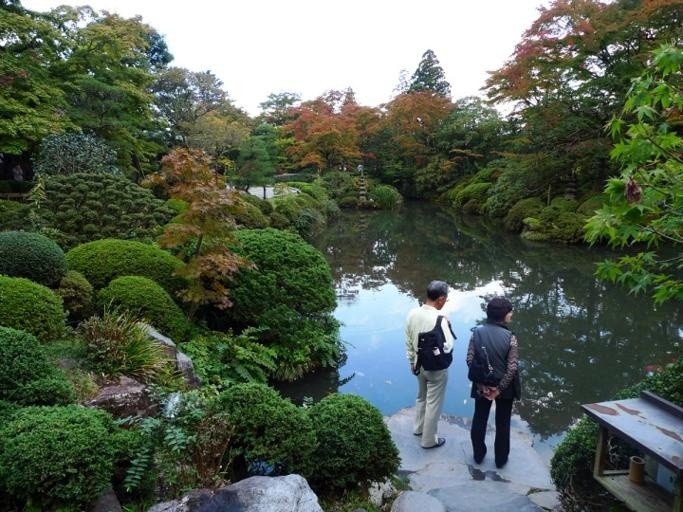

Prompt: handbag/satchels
417;330;453;371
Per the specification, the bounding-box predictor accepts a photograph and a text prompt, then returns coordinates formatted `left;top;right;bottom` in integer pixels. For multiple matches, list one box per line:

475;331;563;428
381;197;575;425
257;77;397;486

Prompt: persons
465;296;521;468
404;279;454;449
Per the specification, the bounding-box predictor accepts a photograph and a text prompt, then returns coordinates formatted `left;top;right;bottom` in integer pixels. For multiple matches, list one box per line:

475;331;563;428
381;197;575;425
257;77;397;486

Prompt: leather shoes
421;438;445;449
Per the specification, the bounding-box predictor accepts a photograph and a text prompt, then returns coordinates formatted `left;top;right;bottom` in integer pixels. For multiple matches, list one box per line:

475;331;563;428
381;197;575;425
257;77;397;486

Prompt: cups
433;347;440;355
628;455;645;481
